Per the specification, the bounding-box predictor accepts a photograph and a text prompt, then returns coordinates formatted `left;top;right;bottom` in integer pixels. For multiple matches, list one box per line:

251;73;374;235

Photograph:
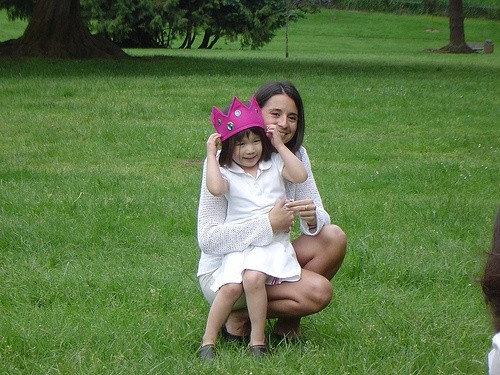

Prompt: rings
305;205;309;211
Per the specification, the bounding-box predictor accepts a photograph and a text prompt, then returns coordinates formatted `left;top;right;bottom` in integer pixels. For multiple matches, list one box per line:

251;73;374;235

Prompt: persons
479;209;500;375
196;82;347;347
197;94;308;365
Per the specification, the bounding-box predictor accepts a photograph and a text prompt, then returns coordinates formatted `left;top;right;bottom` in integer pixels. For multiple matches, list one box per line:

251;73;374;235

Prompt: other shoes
249;343;269;358
198;345;215;361
273;333;305;346
221;323;249;345
